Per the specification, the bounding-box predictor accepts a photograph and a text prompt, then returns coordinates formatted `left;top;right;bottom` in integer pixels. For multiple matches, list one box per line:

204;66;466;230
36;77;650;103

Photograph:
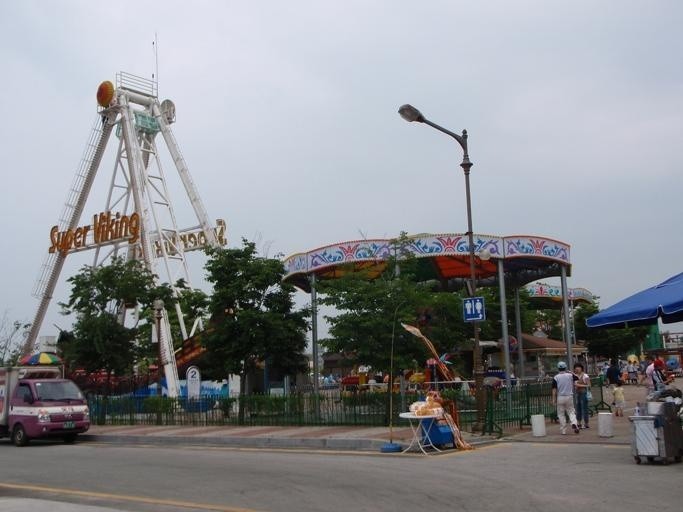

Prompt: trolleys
628;412;683;464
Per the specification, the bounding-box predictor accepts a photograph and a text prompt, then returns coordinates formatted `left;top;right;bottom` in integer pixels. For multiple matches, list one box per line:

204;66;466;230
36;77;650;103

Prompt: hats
557;361;567;368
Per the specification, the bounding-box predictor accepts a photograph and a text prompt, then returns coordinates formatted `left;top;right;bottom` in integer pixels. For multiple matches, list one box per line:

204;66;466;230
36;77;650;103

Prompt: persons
612;381;624;416
552;361;580;435
573;351;676;394
425;391;473;450
317;373;346;384
572;363;591;429
383;372;390;383
219;379;230;417
397;368;404;377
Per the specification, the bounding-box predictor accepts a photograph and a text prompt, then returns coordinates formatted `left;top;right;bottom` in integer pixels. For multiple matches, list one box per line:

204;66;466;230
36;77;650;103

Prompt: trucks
0;365;90;446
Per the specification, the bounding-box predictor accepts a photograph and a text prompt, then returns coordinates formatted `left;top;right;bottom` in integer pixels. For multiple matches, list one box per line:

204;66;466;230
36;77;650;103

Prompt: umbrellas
585;271;683;329
21;352;63;365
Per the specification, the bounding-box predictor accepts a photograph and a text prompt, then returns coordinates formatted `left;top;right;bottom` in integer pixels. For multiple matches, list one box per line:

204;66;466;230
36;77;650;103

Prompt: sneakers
572;424;579;433
578;424;590;429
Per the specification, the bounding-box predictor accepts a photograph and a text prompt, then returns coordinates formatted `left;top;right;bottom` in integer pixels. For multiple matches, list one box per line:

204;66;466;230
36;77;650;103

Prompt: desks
399;412;442;455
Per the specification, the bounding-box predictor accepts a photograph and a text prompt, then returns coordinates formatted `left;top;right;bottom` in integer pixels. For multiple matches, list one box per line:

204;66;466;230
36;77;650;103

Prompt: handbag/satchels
586;385;593;401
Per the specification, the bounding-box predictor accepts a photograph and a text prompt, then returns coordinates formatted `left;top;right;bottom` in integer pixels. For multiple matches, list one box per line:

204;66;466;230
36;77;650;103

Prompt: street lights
397;103;489;435
